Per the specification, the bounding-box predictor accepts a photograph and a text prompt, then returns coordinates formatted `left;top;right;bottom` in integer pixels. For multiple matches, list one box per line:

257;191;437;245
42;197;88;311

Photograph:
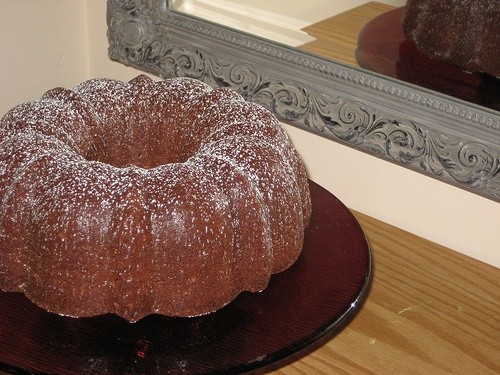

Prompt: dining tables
232;207;500;375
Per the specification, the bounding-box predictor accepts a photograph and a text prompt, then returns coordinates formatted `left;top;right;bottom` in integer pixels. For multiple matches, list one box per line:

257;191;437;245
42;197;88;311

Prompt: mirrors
105;1;500;205
